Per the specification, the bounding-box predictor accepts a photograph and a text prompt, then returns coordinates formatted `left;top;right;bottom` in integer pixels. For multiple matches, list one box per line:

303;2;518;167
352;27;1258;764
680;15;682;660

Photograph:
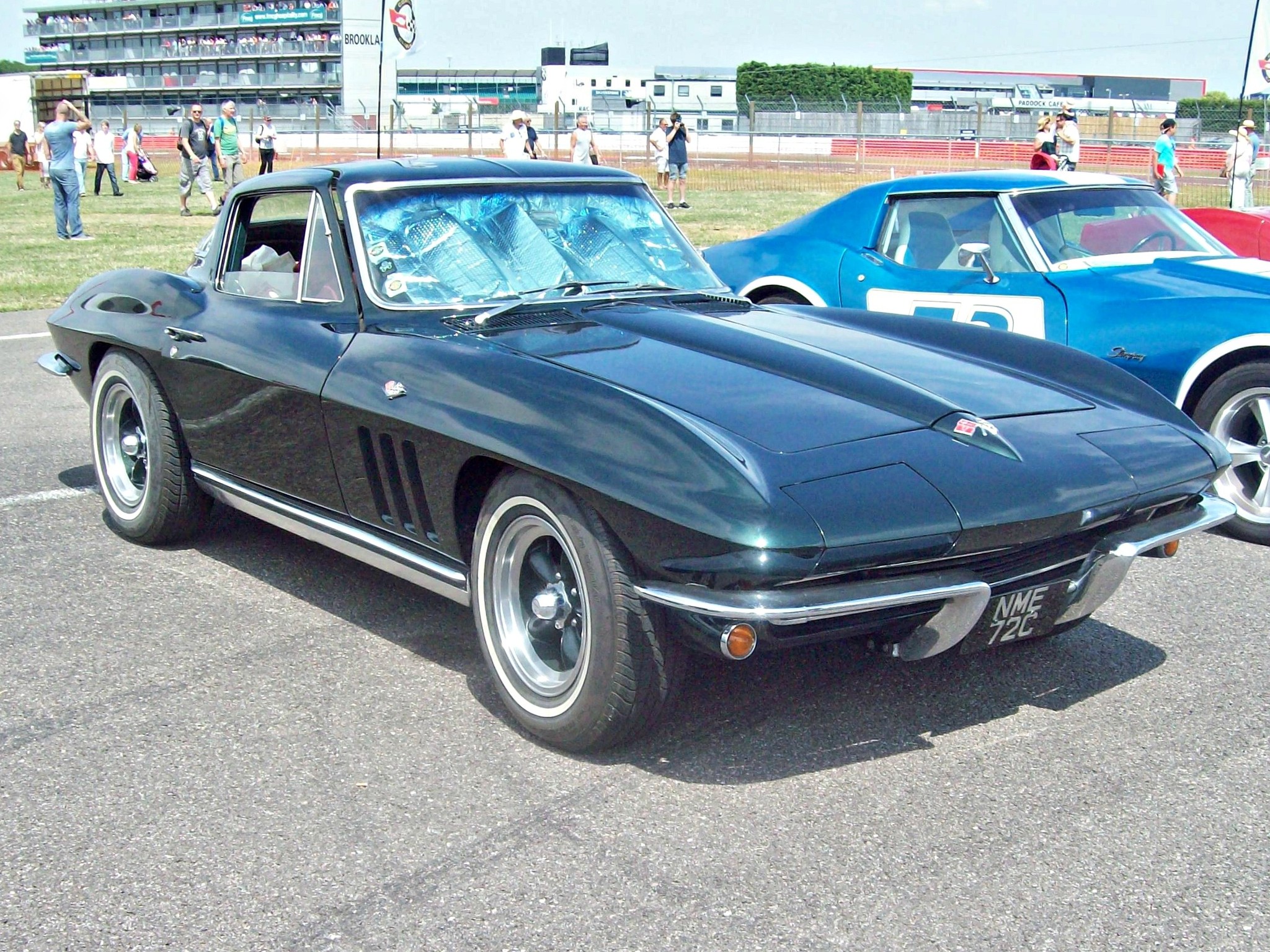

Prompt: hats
1229;119;1257;141
510;110;526;121
265;116;271;121
1036;98;1077;131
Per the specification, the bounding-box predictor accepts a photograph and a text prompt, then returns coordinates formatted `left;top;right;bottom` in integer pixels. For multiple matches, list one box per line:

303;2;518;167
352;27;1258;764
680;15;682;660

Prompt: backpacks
177;119;206;151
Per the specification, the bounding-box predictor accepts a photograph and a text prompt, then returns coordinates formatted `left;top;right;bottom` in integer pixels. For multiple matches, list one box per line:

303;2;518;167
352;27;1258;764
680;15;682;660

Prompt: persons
23;0;344;87
42;99;96;241
5;97;691;215
1033;98;1260;212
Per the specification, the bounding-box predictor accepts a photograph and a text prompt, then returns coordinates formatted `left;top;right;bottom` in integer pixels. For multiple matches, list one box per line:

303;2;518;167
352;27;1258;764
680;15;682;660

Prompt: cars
700;168;1269;547
31;157;1238;753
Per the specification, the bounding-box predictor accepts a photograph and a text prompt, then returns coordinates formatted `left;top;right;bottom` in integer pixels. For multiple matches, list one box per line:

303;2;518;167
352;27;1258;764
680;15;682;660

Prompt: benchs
242;219;350;261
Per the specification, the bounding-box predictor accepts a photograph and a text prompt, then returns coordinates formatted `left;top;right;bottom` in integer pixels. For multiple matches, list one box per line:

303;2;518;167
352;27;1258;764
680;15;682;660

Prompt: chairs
988;211;1065;272
894;209;983;271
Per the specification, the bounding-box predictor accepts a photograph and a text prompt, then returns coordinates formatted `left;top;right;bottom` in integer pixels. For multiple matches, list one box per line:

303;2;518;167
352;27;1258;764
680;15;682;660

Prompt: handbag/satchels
590;155;598;165
255;124;263;144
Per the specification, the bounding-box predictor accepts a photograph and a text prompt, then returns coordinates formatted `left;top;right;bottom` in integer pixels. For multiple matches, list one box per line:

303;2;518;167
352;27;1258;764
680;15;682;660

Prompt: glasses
193;111;201;113
662;124;668;126
1045;121;1049;126
266;119;272;121
527;120;532;122
1056;119;1063;122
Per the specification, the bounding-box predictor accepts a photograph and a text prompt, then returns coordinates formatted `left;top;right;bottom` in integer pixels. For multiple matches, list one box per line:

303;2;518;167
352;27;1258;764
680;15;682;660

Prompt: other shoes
80;193;86;197
215;178;223;181
180;208;192;216
212;206;221;216
128;180;141;184
113;192;123;196
218;196;224;206
94;190;99;196
19;188;24;191
679;202;692;209
71;234;95;241
667;202;674;209
